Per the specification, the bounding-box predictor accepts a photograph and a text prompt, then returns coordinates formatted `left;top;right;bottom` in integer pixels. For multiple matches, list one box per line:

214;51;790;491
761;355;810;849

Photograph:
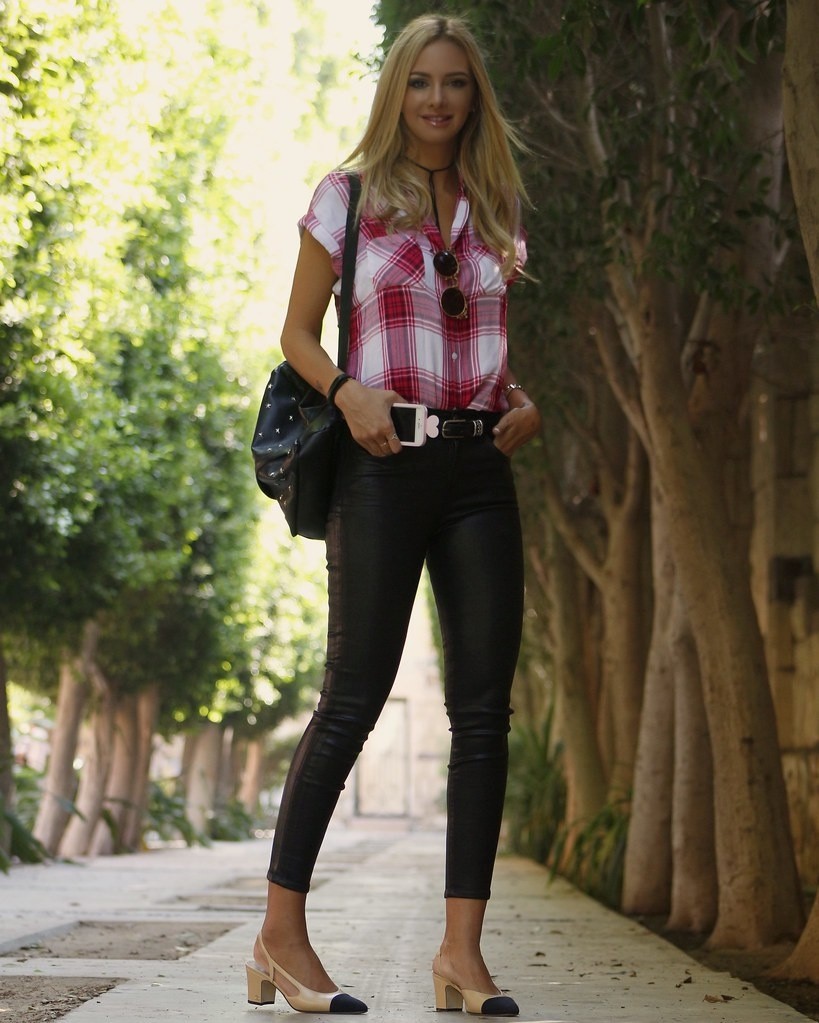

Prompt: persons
244;11;543;1015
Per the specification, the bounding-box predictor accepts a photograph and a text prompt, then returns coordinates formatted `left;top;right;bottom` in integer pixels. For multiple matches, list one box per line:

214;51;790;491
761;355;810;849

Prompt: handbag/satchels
251;360;342;540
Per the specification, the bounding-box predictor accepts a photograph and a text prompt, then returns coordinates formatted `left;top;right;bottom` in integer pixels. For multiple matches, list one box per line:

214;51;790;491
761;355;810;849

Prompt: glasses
433;250;469;320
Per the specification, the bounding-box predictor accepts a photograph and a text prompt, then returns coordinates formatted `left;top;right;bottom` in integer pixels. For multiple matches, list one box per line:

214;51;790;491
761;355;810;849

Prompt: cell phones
391;403;427;446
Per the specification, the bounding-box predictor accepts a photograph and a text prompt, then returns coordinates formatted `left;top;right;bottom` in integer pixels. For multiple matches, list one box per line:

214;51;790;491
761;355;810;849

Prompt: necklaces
401;148;458;235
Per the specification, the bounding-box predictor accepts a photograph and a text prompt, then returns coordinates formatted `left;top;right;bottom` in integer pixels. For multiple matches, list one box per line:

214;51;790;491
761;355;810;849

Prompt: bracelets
326;371;355;407
505;383;525;402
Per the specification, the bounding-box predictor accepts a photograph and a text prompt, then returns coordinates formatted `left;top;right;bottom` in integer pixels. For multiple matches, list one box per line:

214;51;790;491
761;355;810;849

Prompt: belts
427;413;499;440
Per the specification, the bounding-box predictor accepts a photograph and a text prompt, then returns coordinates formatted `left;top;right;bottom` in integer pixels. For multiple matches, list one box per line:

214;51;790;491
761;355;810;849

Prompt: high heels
432;952;519;1016
245;935;369;1013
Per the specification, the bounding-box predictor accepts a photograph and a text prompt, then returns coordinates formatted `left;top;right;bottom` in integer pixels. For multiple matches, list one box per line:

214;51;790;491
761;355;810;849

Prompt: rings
391;433;398;440
380;440;387;448
